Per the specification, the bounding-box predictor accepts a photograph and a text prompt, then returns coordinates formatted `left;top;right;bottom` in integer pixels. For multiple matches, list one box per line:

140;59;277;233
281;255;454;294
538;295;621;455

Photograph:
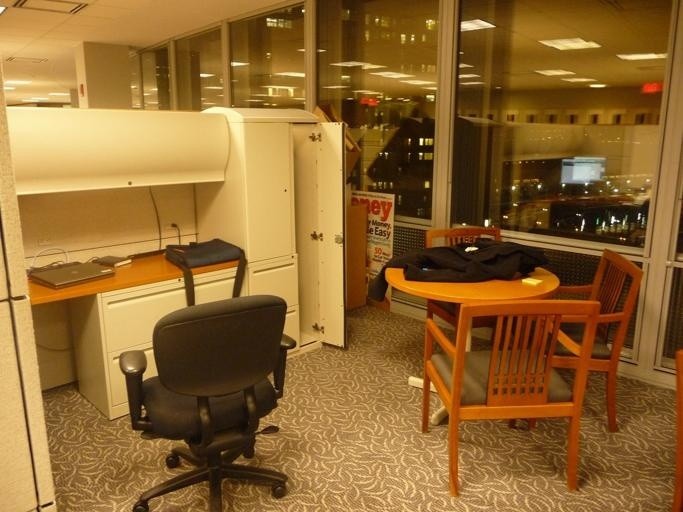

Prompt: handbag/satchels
165;238;246;268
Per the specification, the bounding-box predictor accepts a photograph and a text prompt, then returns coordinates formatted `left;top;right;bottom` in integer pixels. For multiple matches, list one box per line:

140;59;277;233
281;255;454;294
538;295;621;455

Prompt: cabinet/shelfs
196;107;348;358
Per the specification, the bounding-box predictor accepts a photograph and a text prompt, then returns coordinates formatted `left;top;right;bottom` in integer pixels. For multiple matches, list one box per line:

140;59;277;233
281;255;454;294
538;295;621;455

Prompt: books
91;255;133;268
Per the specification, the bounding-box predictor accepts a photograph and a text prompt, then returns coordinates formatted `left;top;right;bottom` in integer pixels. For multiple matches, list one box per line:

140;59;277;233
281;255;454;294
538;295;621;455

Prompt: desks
5;107;247;420
385;266;560;425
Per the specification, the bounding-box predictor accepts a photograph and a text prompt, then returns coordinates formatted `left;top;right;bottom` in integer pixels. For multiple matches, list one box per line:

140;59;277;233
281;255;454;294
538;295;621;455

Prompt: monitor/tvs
560;157;594;197
573;155;608;181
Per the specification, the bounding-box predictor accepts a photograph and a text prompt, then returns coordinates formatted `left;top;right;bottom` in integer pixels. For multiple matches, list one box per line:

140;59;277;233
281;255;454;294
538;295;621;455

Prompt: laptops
29;262;116;289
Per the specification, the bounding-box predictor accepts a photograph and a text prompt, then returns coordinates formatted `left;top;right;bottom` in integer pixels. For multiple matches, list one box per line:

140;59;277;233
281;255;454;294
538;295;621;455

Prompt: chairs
538;248;644;432
424;227;500;329
118;297;297;512
671;351;683;512
424;299;599;496
